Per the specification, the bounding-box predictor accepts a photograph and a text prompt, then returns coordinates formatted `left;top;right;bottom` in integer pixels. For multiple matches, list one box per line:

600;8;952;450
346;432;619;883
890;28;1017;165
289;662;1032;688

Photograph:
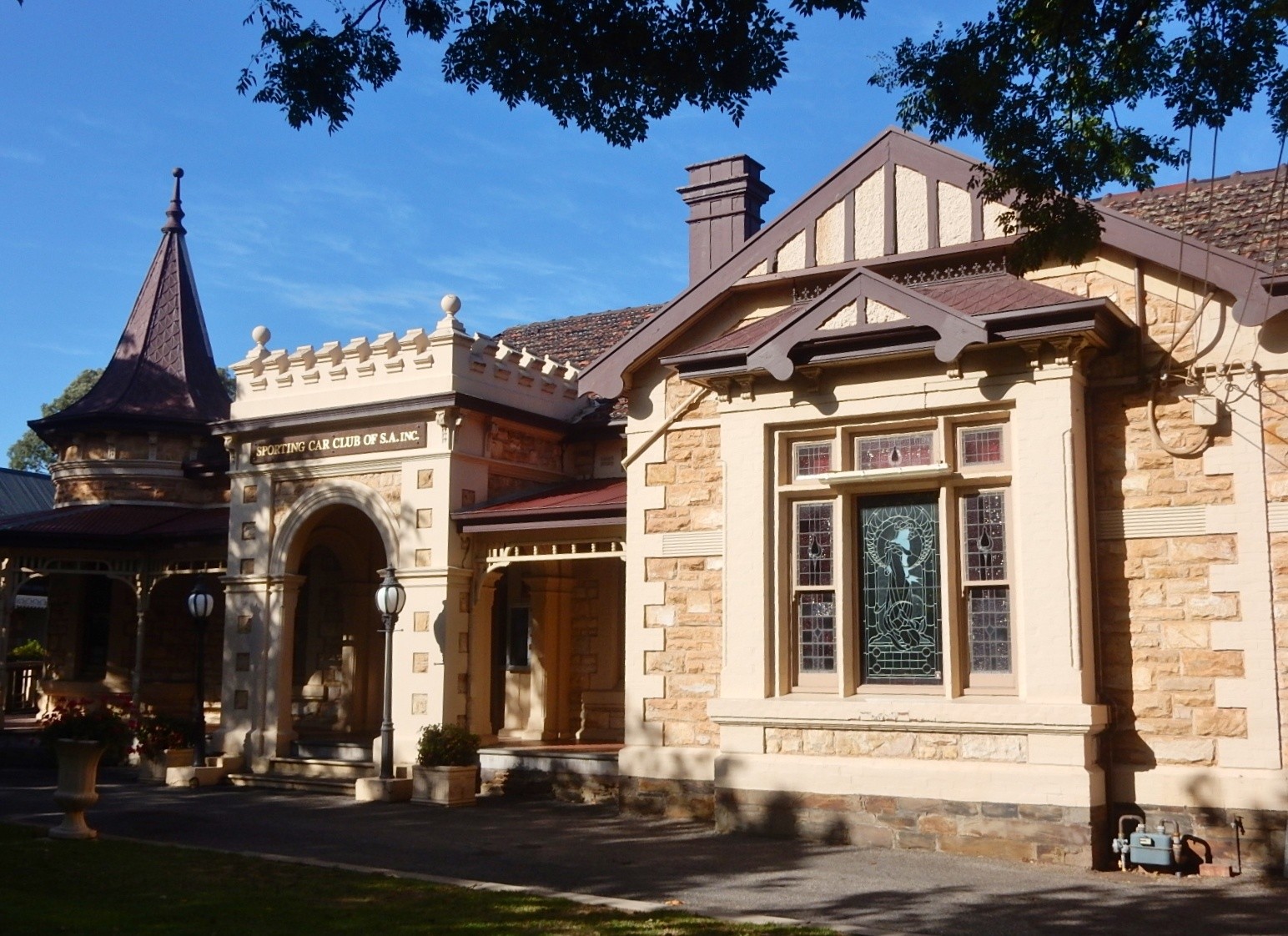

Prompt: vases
41;729;111;839
411;764;481;808
133;747;194;780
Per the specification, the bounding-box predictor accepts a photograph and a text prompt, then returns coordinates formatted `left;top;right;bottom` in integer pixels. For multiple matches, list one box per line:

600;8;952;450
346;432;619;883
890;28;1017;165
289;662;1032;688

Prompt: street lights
186;570;213;764
375;561;408;779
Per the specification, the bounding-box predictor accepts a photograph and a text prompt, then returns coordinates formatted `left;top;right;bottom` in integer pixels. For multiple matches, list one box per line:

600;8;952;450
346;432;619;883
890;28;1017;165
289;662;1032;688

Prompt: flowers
43;689;119;749
418;724;480;767
106;698;183;765
